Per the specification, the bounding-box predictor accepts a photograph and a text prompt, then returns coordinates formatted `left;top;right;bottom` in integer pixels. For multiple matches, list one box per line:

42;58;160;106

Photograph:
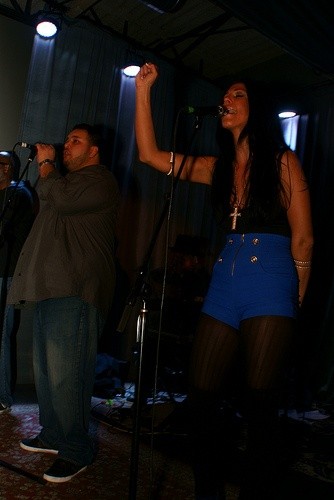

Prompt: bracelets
294;260;312;269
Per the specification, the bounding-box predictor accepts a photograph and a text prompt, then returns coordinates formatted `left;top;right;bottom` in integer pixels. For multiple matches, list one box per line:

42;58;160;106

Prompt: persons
7;126;111;482
136;63;314;492
0;151;38;415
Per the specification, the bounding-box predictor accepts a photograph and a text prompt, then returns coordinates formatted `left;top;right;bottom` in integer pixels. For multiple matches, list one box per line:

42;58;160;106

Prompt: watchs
38;159;55;167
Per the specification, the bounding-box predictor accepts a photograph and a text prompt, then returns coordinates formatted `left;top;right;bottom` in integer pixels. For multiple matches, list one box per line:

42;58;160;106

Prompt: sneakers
20;436;59;455
43;448;88;484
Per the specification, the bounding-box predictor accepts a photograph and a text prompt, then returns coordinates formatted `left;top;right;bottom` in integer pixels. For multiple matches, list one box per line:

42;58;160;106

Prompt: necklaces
231;167;250;228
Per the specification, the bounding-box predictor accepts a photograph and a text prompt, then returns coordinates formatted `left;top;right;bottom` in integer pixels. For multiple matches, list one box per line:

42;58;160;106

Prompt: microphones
17;142;63;153
181;104;226;116
